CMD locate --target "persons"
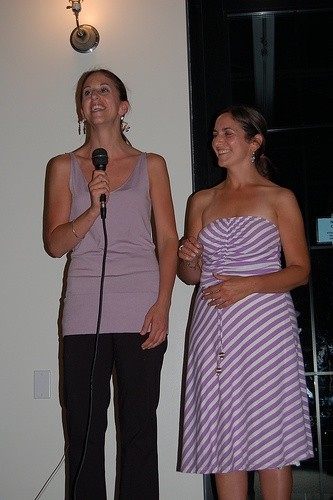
[43,69,181,500]
[176,107,314,500]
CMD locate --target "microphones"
[92,148,108,220]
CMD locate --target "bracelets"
[72,220,85,240]
[184,260,199,268]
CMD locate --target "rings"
[179,245,184,251]
